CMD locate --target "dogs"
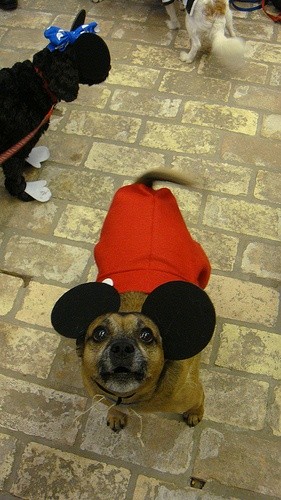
[0,32,111,202]
[51,170,216,432]
[162,0,244,64]
